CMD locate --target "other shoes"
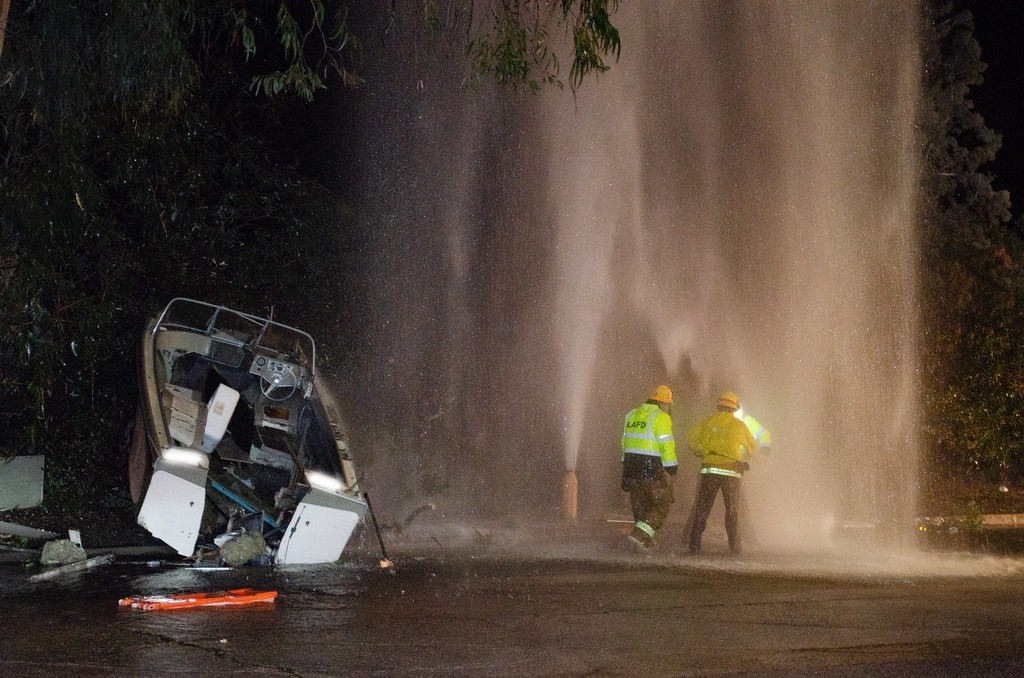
[626,535,650,554]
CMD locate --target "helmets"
[648,386,674,404]
[716,390,741,410]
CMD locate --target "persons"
[685,390,757,556]
[619,384,679,559]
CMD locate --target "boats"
[126,296,363,563]
